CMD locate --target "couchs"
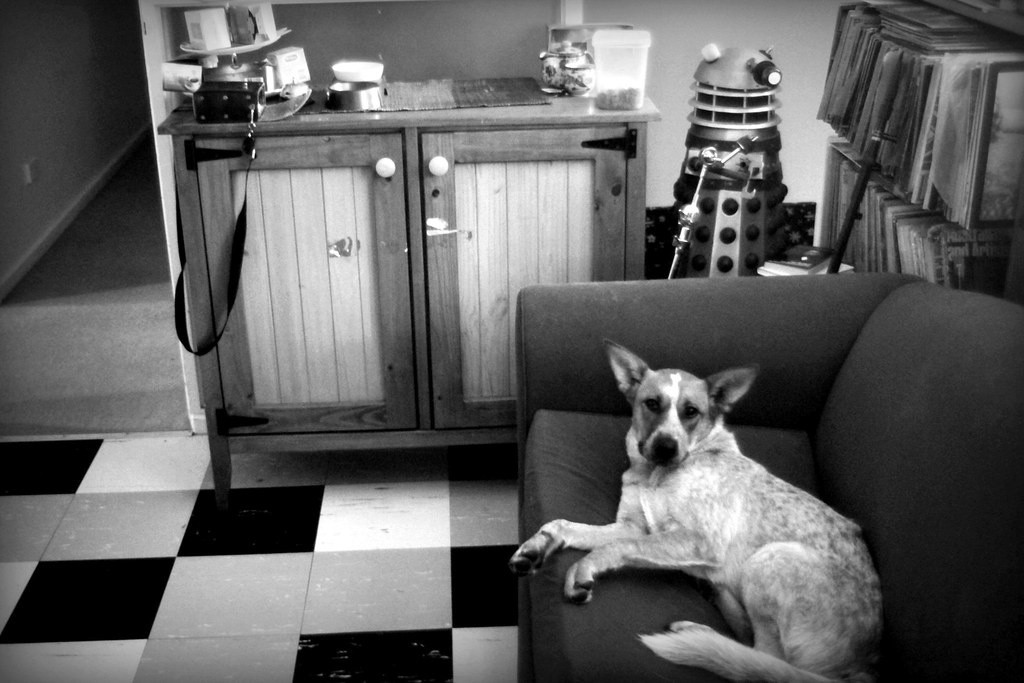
[511,278,1024,683]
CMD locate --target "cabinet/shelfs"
[158,76,660,486]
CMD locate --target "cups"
[564,62,596,97]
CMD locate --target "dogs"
[510,336,887,683]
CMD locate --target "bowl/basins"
[332,61,385,82]
[324,80,379,110]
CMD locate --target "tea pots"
[539,41,594,94]
